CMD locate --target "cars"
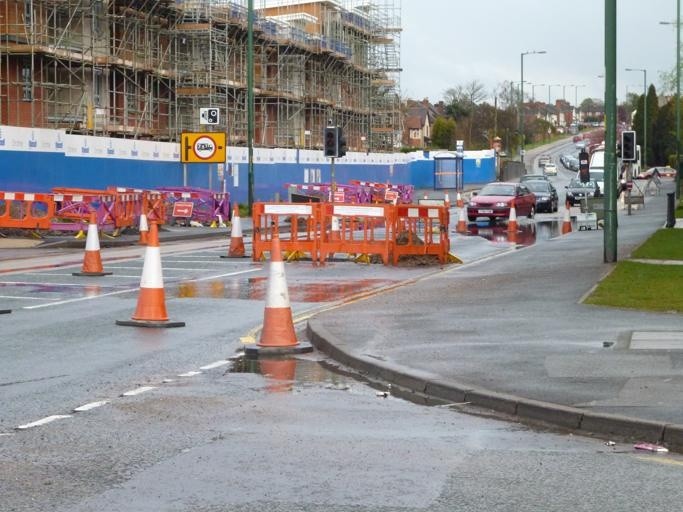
[465,179,537,223]
[589,120,605,128]
[465,219,538,250]
[518,131,676,216]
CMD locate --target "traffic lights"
[336,126,346,159]
[324,126,338,158]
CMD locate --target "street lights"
[656,20,680,163]
[624,68,649,171]
[507,79,589,144]
[596,74,605,79]
[518,49,551,161]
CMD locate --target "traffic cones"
[500,200,523,235]
[215,197,253,259]
[134,206,155,244]
[559,200,573,235]
[247,349,299,395]
[239,230,318,353]
[420,188,469,234]
[114,216,187,329]
[68,209,113,278]
